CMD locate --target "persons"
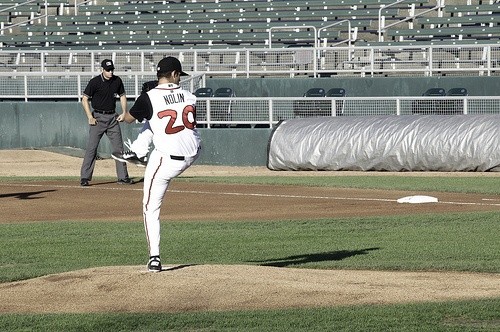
[112,57,201,273]
[81,58,134,186]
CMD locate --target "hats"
[102,59,114,72]
[156,56,189,78]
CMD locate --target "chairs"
[294,88,345,116]
[192,88,234,129]
[412,88,467,115]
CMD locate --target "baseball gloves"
[141,81,159,94]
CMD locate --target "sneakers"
[110,150,146,166]
[147,255,161,271]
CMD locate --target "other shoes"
[118,178,133,184]
[81,179,89,186]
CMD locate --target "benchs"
[0,0,500,78]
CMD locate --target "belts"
[96,110,113,114]
[170,156,185,160]
